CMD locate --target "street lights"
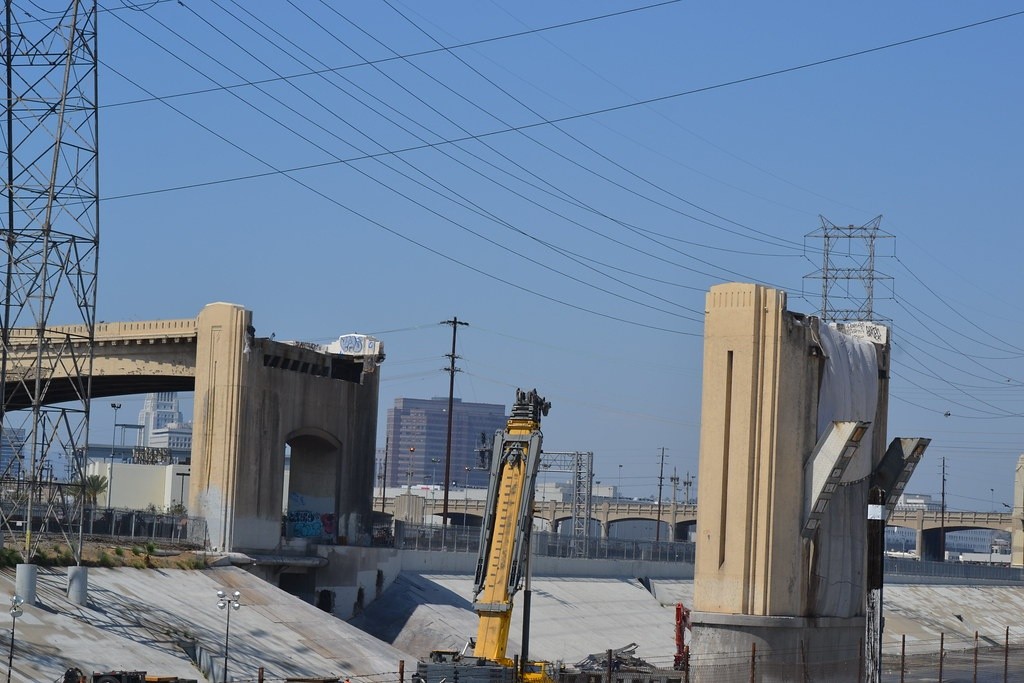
[464,466,471,553]
[990,489,994,512]
[617,465,623,503]
[428,458,441,551]
[594,479,599,558]
[407,447,415,522]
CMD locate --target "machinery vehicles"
[412,386,559,683]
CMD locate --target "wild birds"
[944,411,950,418]
[1002,503,1009,507]
